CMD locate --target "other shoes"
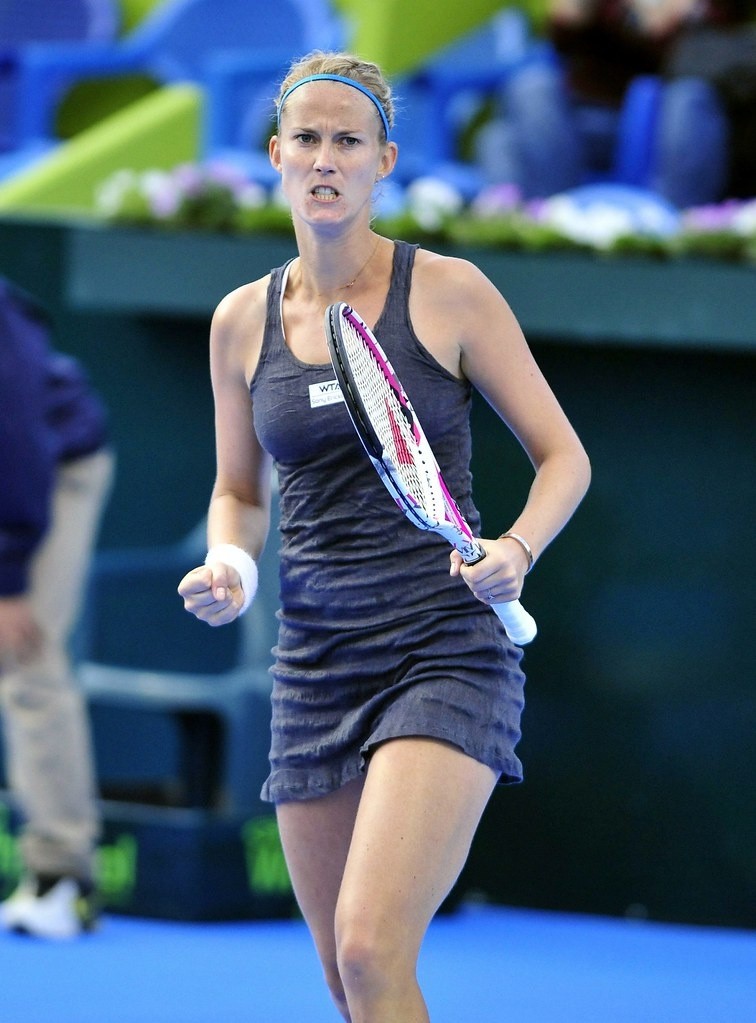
[0,870,85,938]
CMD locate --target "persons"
[0,284,121,940]
[177,55,593,1023]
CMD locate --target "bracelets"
[205,543,260,617]
[499,533,536,574]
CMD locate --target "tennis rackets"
[322,299,539,644]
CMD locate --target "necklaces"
[298,237,381,291]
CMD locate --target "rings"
[488,588,495,603]
